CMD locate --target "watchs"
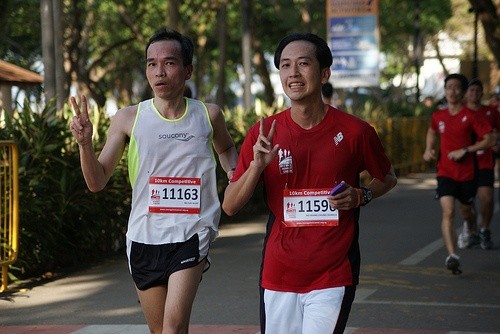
[360,186,373,207]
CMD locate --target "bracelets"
[354,188,360,208]
[463,147,469,153]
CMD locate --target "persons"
[322,82,340,110]
[463,78,500,251]
[222,34,397,334]
[423,73,493,275]
[69,26,239,334]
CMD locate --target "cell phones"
[330,183,346,197]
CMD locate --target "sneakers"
[446,254,464,276]
[480,227,492,249]
[457,220,474,250]
